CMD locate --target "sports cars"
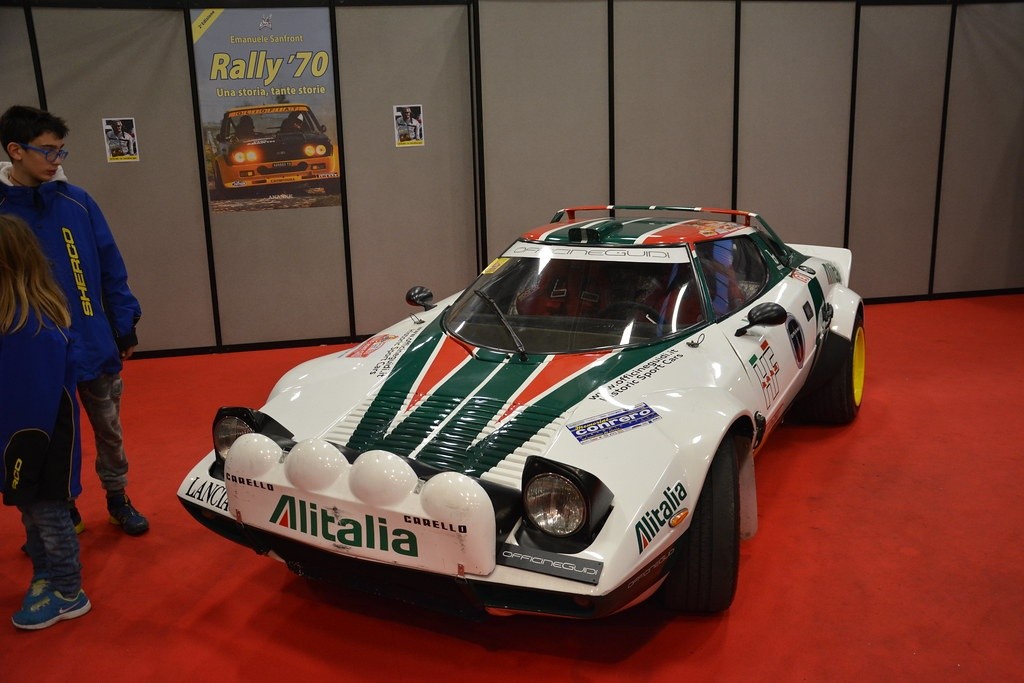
[179,205,865,629]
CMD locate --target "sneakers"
[20,573,49,609]
[106,493,148,533]
[21,508,85,553]
[11,590,91,631]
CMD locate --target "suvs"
[206,102,342,197]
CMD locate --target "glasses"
[20,145,68,162]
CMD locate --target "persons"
[0,214,92,629]
[396,107,420,141]
[280,111,305,134]
[106,120,134,157]
[0,105,150,558]
[234,115,256,141]
